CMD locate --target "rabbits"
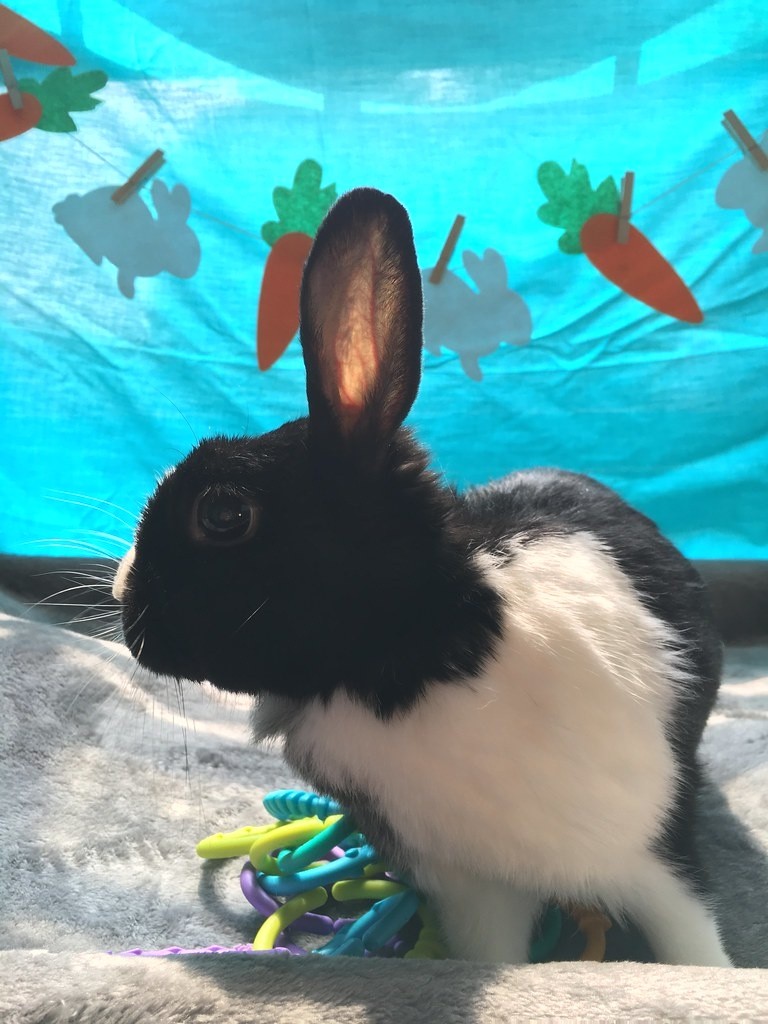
[101,188,738,968]
